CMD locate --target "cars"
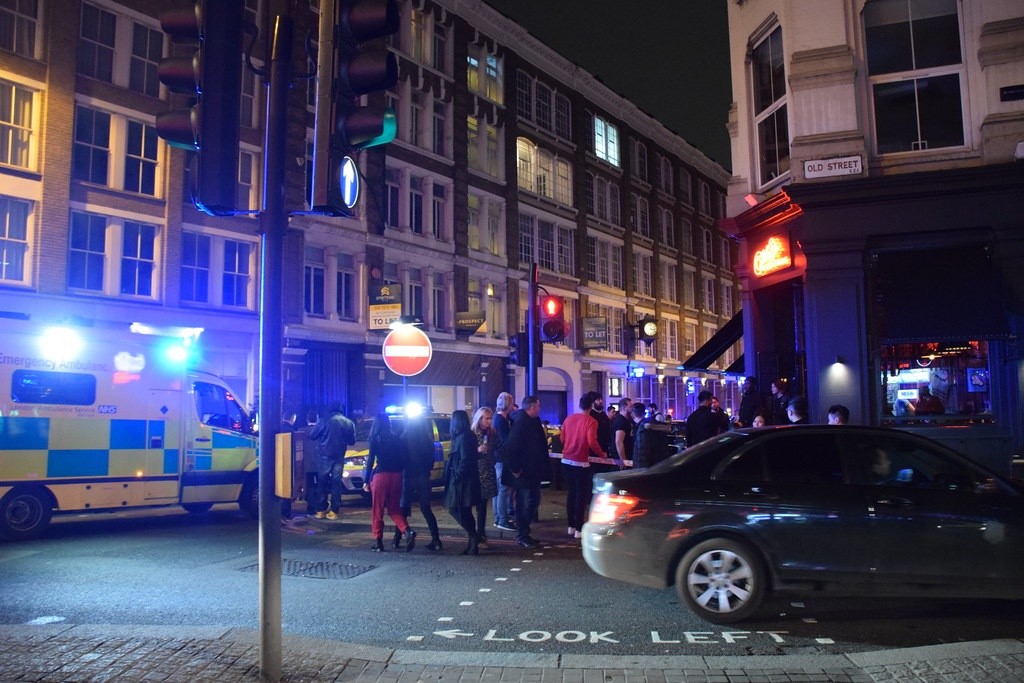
[340,413,452,505]
[581,423,1024,626]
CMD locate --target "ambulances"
[0,332,260,541]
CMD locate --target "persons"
[895,392,915,416]
[767,379,794,425]
[738,376,764,428]
[786,399,808,424]
[441,391,551,557]
[560,396,607,539]
[687,391,729,448]
[750,408,773,428]
[826,405,850,425]
[362,409,443,552]
[278,400,356,519]
[915,386,944,415]
[584,391,671,509]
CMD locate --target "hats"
[588,391,602,403]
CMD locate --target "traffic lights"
[541,294,566,343]
[157,0,244,217]
[326,0,399,220]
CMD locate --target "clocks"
[644,322,657,336]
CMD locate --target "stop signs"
[382,326,432,377]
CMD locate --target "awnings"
[676,308,745,377]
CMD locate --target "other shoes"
[406,530,416,551]
[371,543,383,551]
[519,540,539,548]
[567,527,576,535]
[425,540,442,550]
[326,512,336,519]
[574,530,581,539]
[494,522,519,531]
[391,541,398,549]
[285,517,295,522]
[315,511,323,519]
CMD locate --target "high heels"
[459,544,479,554]
[469,531,485,552]
